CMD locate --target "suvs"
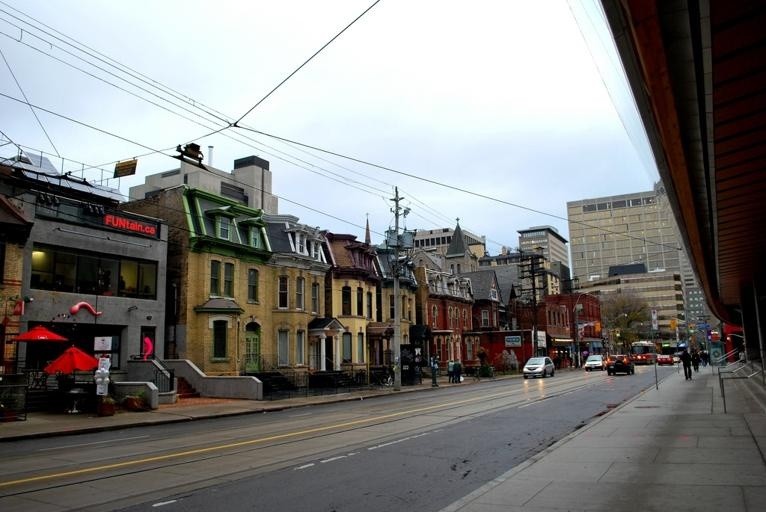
[607,355,634,376]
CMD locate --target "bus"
[699,343,708,354]
[629,338,689,366]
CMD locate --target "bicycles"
[379,370,394,387]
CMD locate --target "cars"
[584,355,606,371]
[523,357,555,378]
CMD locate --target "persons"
[553,354,587,371]
[448,355,481,383]
[680,348,708,380]
[739,350,745,366]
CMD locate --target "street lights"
[614,313,627,322]
[570,290,591,367]
[392,247,437,390]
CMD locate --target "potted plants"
[98,397,116,415]
[2,390,25,421]
[126,388,146,408]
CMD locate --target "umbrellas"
[44,347,99,389]
[12,324,69,370]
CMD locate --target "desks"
[20,367,88,413]
